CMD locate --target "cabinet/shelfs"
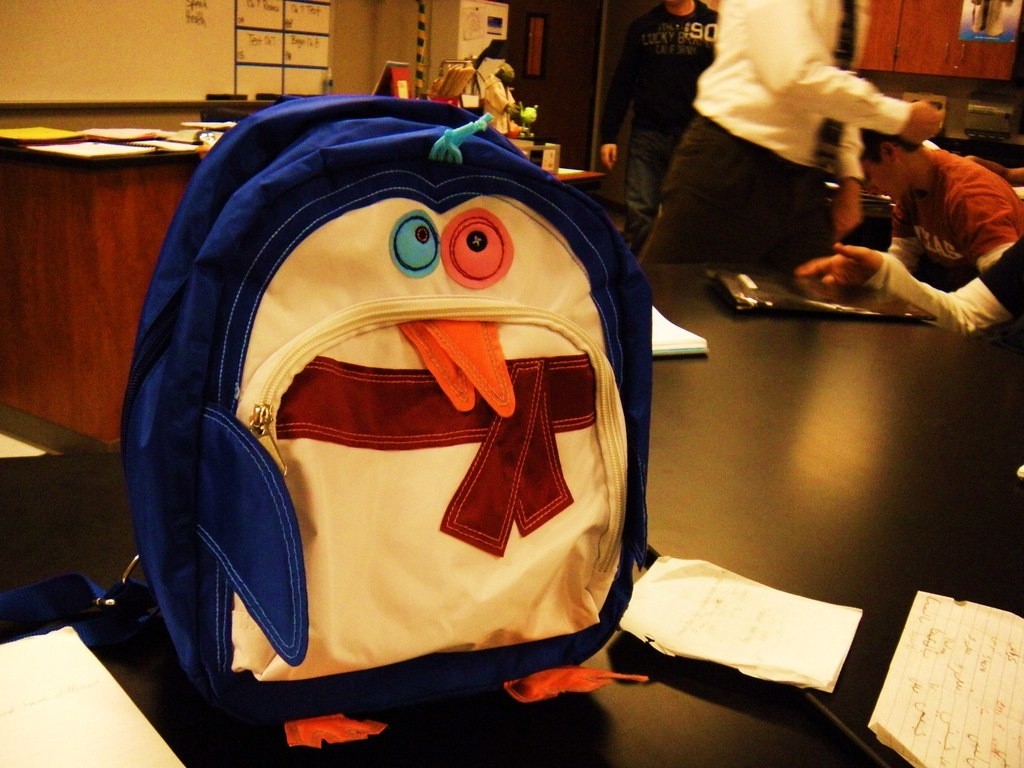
[858,0,1023,81]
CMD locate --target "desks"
[0,140,208,456]
[0,264,1024,768]
[534,166,604,183]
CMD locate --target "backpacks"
[0,97,651,746]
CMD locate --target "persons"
[600,0,719,252]
[484,63,521,134]
[795,128,1024,341]
[639,1,944,266]
